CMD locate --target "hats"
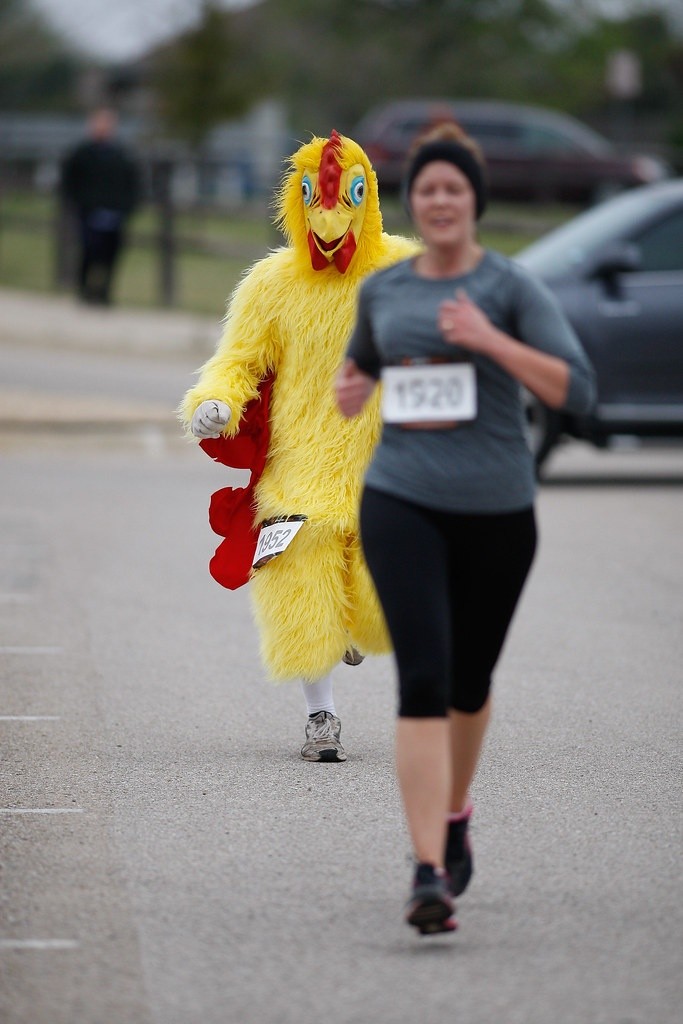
[401,120,490,220]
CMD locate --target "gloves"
[189,398,231,439]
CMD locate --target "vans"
[511,180,683,470]
[355,100,665,211]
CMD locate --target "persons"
[333,134,592,935]
[409,112,489,220]
[57,109,145,305]
[181,130,423,760]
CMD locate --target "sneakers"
[301,711,346,762]
[445,797,472,897]
[342,641,365,666]
[401,863,457,934]
